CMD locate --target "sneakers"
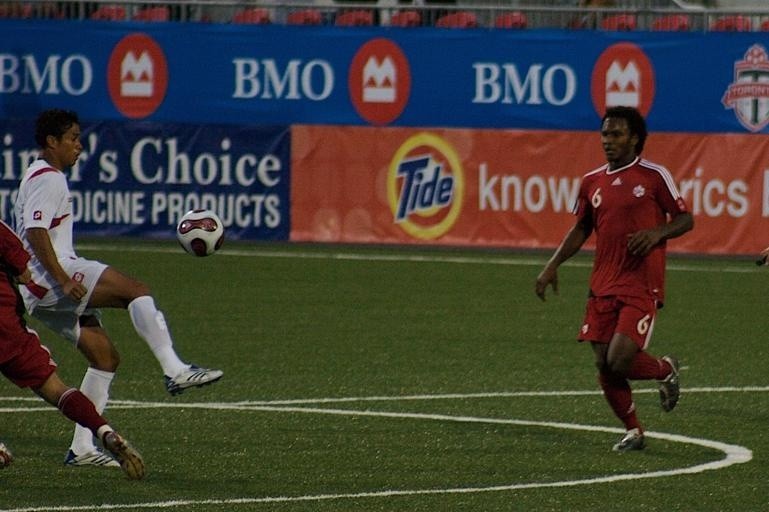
[102,430,145,479]
[613,431,646,451]
[658,354,680,412]
[63,449,122,468]
[164,365,224,395]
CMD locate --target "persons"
[536,104,694,453]
[0,217,146,479]
[17,108,224,469]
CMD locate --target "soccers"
[176,208,225,257]
